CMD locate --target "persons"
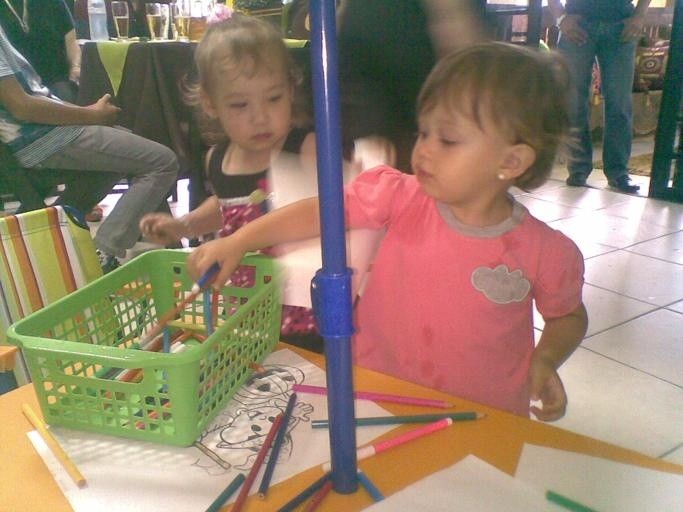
[546,1,655,194]
[336,0,493,175]
[171,36,592,429]
[0,26,180,272]
[0,0,85,91]
[133,13,325,352]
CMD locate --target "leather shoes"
[607,176,640,192]
[565,171,588,187]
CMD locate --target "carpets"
[592,147,682,183]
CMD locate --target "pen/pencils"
[87,262,265,432]
[203,385,488,512]
[21,402,86,488]
[546,490,595,512]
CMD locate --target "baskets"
[4,247,282,449]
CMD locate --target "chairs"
[1,142,124,391]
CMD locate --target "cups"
[174,0,215,43]
[110,2,130,40]
[145,3,170,41]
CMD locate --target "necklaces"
[4,1,29,32]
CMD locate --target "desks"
[77,39,311,165]
[0,312,683,511]
